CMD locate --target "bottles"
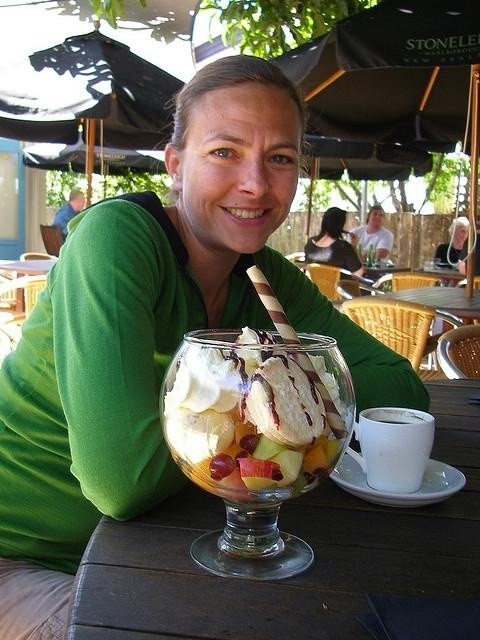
[357,240,376,268]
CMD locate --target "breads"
[234,327,326,447]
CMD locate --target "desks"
[297,261,374,307]
[380,283,477,319]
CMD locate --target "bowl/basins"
[419,257,442,267]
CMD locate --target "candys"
[246,264,348,439]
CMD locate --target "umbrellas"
[266,0,480,324]
[1,20,186,207]
[21,141,167,199]
[299,138,434,233]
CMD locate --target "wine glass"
[157,327,360,584]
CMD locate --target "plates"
[329,449,466,509]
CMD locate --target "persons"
[448,233,480,289]
[52,191,85,240]
[304,207,364,289]
[346,206,394,295]
[0,54,430,640]
[434,217,469,286]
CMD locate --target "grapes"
[209,454,235,478]
[240,435,259,452]
[235,450,251,471]
[313,467,328,480]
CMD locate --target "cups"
[346,406,435,495]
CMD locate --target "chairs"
[329,276,386,301]
[436,322,480,377]
[338,292,462,378]
[0,224,65,337]
[375,270,444,298]
